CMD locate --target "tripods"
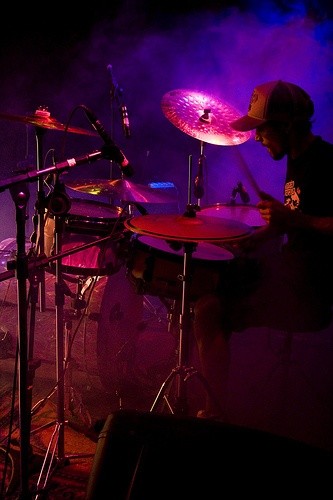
[149,244,227,419]
[30,212,100,500]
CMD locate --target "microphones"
[133,203,181,252]
[51,152,60,188]
[81,105,136,177]
[194,141,206,198]
[120,105,134,136]
[236,181,250,202]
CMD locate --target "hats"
[230,80,314,132]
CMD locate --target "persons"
[195,81,332,419]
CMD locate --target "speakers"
[87,411,333,500]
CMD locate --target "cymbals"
[160,89,250,146]
[123,213,255,243]
[65,176,179,205]
[0,108,98,138]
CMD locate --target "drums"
[126,235,234,297]
[0,236,146,395]
[34,205,124,275]
[198,202,274,235]
